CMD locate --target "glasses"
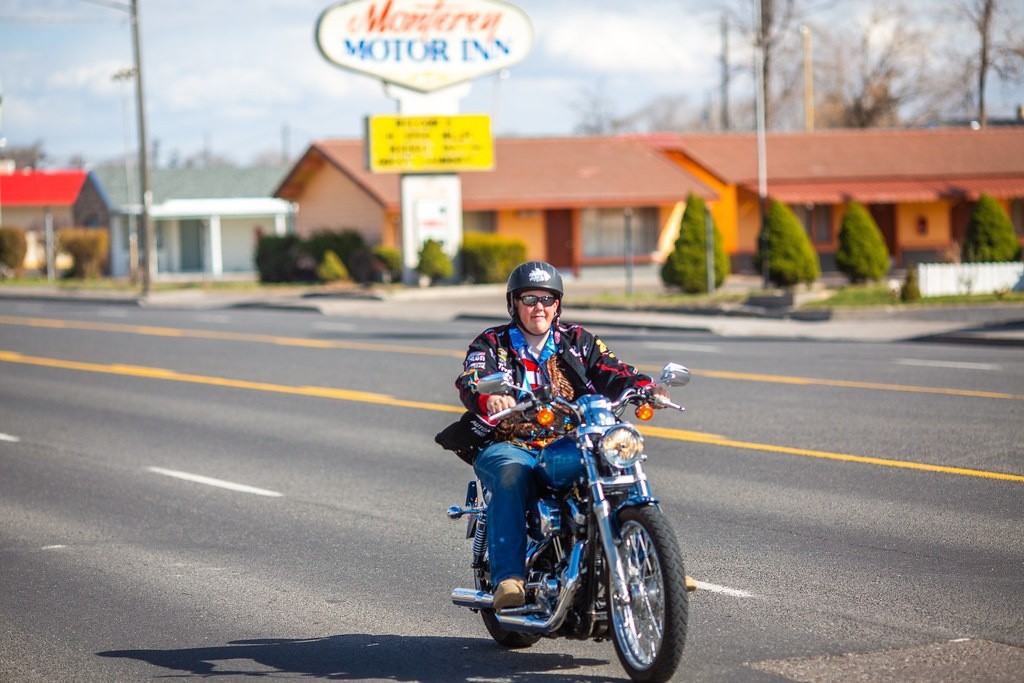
[514,294,556,307]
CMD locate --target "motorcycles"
[445,361,696,683]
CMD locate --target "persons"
[454,261,670,610]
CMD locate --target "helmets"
[506,261,563,303]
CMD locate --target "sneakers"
[494,577,527,611]
[685,575,697,592]
